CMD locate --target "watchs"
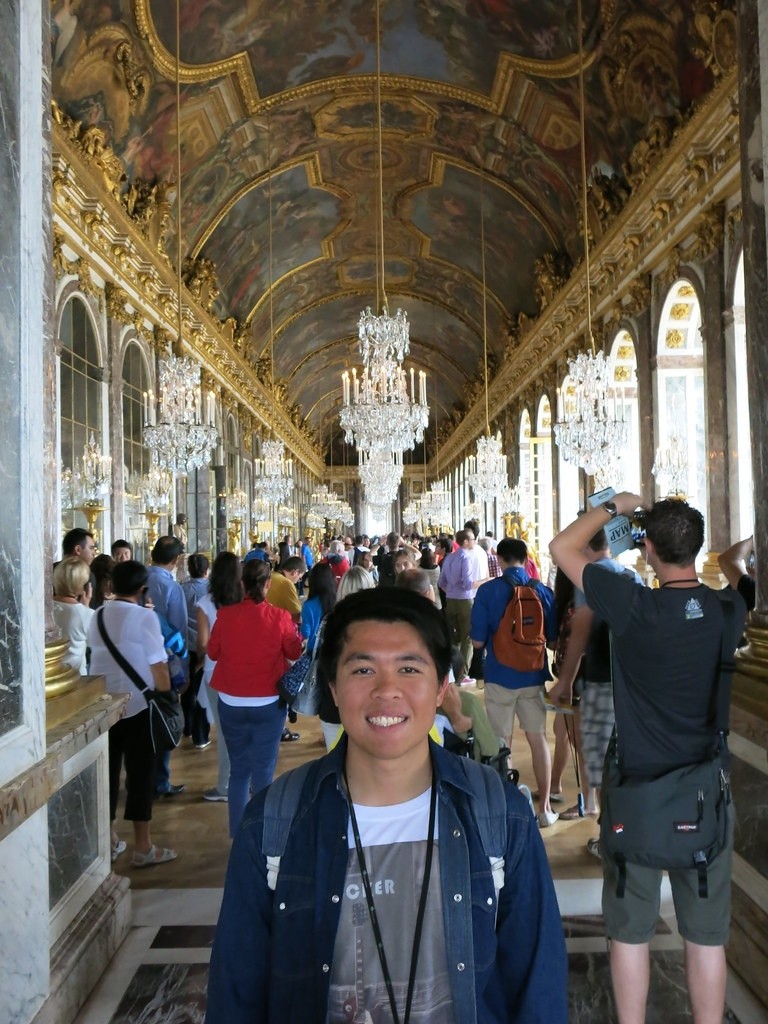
[603,500,617,520]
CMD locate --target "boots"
[453,665,476,686]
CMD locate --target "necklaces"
[114,597,134,603]
[661,578,698,586]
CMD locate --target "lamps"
[550,1,632,479]
[139,465,170,561]
[648,436,694,502]
[224,0,533,554]
[73,437,112,550]
[136,0,219,476]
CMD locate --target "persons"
[207,584,574,1024]
[54,492,756,860]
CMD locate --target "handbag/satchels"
[596,760,734,872]
[279,656,320,718]
[146,693,186,752]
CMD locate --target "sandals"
[111,840,127,864]
[132,845,178,867]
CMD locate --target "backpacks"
[495,576,548,672]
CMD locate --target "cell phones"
[630,511,647,550]
[142,586,149,608]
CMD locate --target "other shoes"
[560,804,599,820]
[156,784,184,799]
[281,730,301,742]
[203,788,228,802]
[588,837,602,858]
[196,740,213,749]
[540,808,560,827]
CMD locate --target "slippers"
[530,790,564,802]
